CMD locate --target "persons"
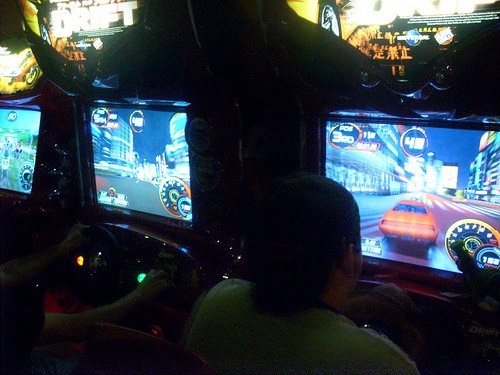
[184,174,500,375]
[0,223,167,375]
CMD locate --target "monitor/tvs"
[0,105,46,200]
[80,99,199,232]
[319,115,500,287]
